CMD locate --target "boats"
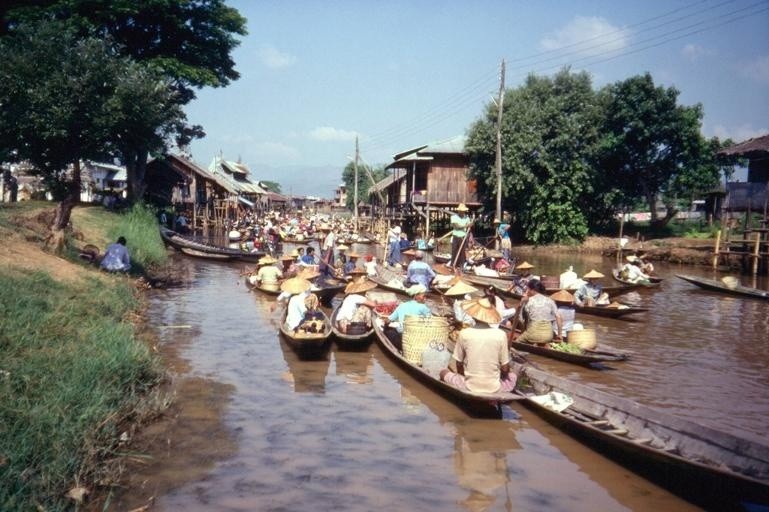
[246,213,650,319]
[279,305,333,350]
[158,222,267,261]
[672,273,769,301]
[499,322,632,366]
[510,363,769,495]
[612,268,663,289]
[329,301,378,344]
[369,313,529,409]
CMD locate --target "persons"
[403,251,436,291]
[440,298,517,392]
[551,290,576,337]
[229,204,356,252]
[494,218,511,260]
[445,276,506,329]
[384,284,431,350]
[99,236,131,273]
[623,250,654,282]
[517,278,562,348]
[573,269,605,307]
[510,261,534,295]
[428,235,434,247]
[451,205,472,272]
[256,223,379,332]
[160,210,167,222]
[386,222,410,266]
[176,215,188,225]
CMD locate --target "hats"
[455,203,470,211]
[583,269,606,279]
[493,218,502,224]
[515,261,535,269]
[487,249,505,258]
[460,298,501,323]
[401,248,479,296]
[258,223,378,294]
[626,253,651,263]
[549,289,578,302]
